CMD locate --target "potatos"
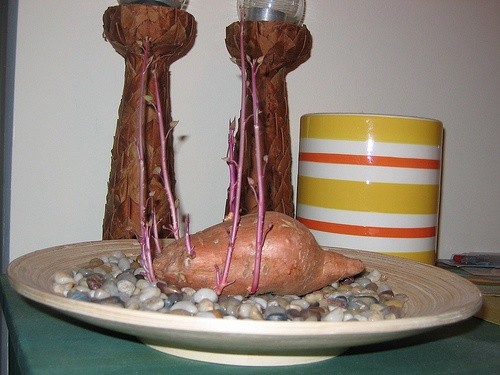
[153,211,366,296]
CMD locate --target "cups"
[295,114,443,267]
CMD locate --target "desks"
[1,274,499,375]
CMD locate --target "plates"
[8,239,483,368]
